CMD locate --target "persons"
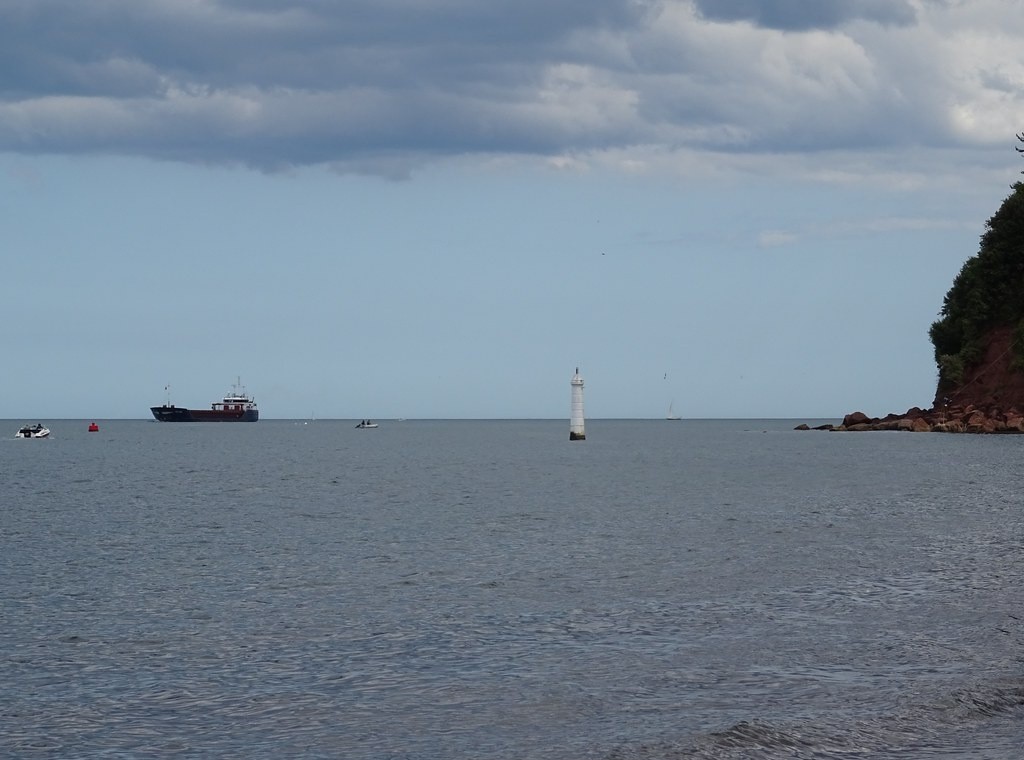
[24,423,44,430]
[361,418,371,425]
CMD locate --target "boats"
[357,424,378,429]
[14,423,50,438]
[150,376,259,422]
